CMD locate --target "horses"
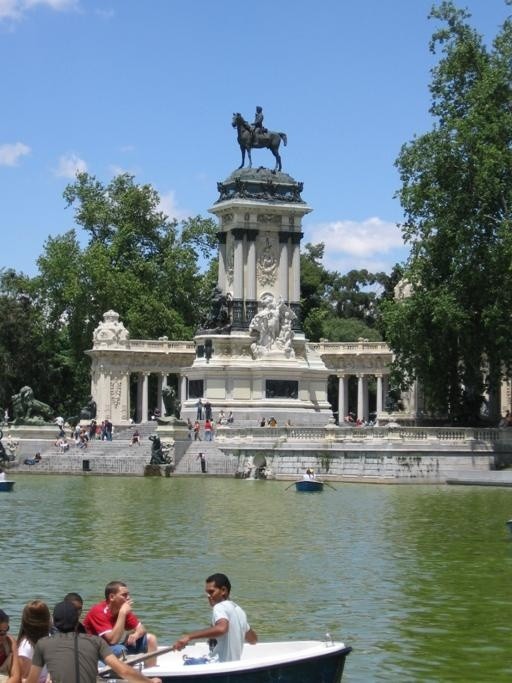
[231,112,288,172]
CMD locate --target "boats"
[97,632,352,682]
[295,479,324,490]
[0,479,15,490]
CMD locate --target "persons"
[331,409,386,429]
[18,600,51,682]
[267,416,277,426]
[63,591,85,632]
[304,465,316,482]
[22,599,163,682]
[194,452,208,473]
[248,105,267,146]
[24,450,43,464]
[154,409,161,419]
[83,579,159,678]
[186,397,235,443]
[258,415,265,428]
[284,418,296,431]
[248,294,298,352]
[48,415,115,451]
[1,607,31,682]
[171,572,258,668]
[148,433,172,464]
[129,429,142,448]
